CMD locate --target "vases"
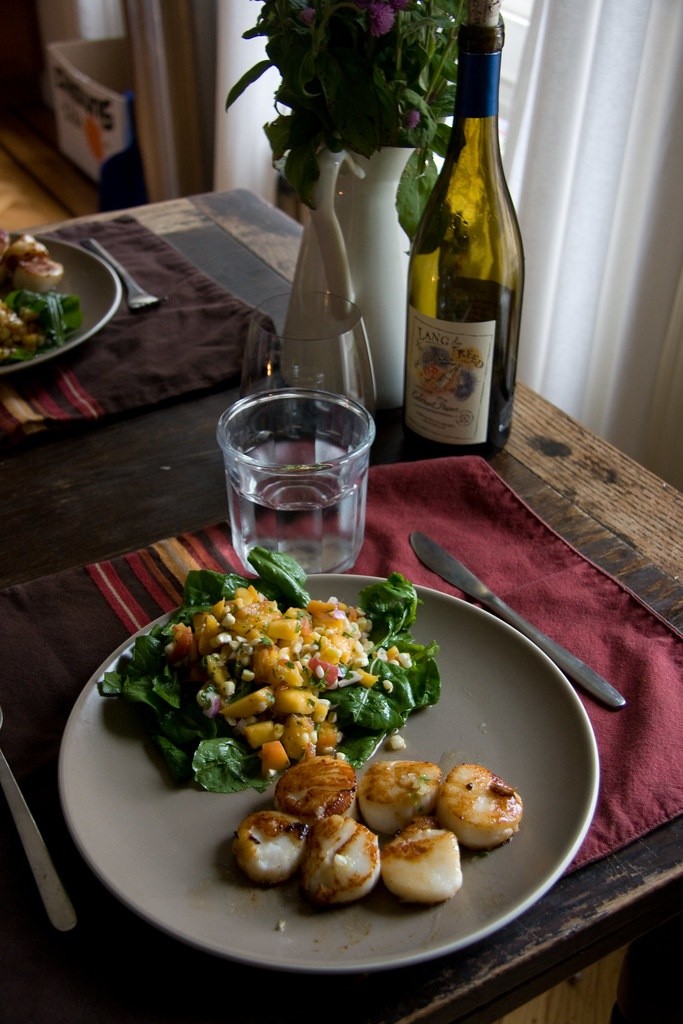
[282,144,413,410]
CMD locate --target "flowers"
[225,1,476,260]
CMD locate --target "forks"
[79,238,169,311]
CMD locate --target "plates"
[0,231,124,373]
[58,570,602,974]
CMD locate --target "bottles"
[399,0,525,456]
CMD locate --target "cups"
[216,385,376,577]
[243,291,379,422]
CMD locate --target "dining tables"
[1,188,683,1023]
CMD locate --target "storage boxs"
[48,34,135,183]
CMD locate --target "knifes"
[398,529,627,702]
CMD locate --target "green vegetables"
[0,287,84,362]
[97,546,441,791]
